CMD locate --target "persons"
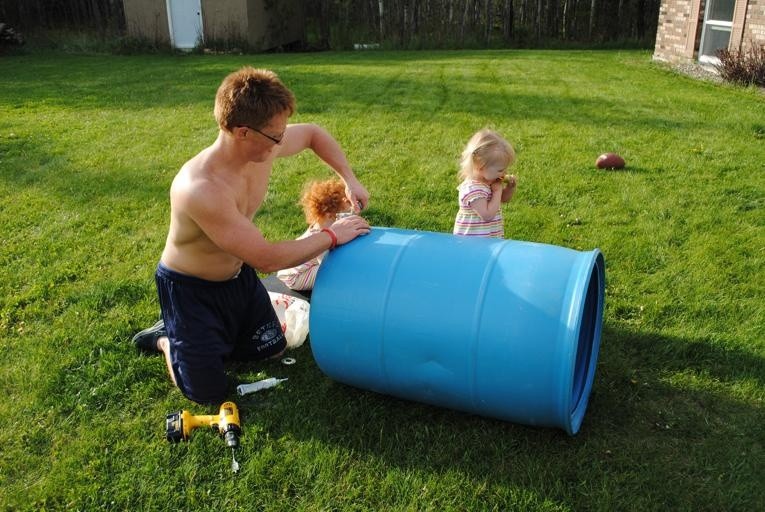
[277,178,350,291]
[451,128,518,238]
[130,67,371,403]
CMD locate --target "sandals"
[131,319,168,356]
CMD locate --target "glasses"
[237,124,286,145]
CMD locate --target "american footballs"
[596,153,625,168]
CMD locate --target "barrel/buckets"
[308,226,605,437]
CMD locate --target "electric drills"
[166,402,242,475]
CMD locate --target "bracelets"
[321,227,337,250]
